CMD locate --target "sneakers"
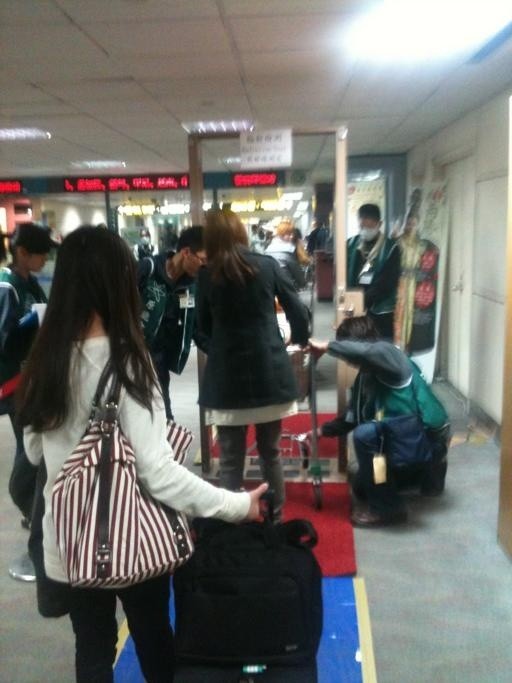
[8,551,36,584]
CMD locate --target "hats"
[13,221,61,255]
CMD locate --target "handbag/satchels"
[27,457,73,619]
[372,410,433,474]
[47,344,195,589]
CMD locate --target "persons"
[0,222,58,530]
[391,188,439,358]
[190,208,314,526]
[10,224,270,683]
[248,214,328,296]
[132,228,155,259]
[127,225,209,422]
[302,316,453,526]
[346,202,400,332]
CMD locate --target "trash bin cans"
[313,250,334,303]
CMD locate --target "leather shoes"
[350,501,409,529]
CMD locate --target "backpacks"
[177,514,324,663]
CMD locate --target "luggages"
[169,631,320,683]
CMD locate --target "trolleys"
[195,339,323,510]
